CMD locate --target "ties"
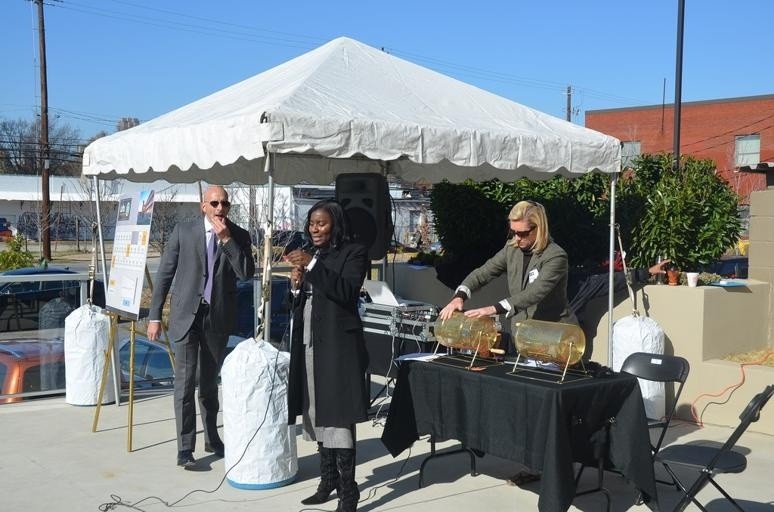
[203,229,216,305]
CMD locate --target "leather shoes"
[178,450,196,466]
[205,444,224,457]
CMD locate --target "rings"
[295,272,296,273]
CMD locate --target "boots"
[334,448,360,512]
[301,446,340,504]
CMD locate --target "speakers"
[336,173,394,260]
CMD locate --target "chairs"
[575,352,690,505]
[654,385,773,511]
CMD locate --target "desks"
[381,353,657,511]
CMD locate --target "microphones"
[295,264,304,289]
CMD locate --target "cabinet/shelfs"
[92,190,176,452]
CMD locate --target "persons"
[147,186,255,467]
[287,199,369,512]
[552,225,670,314]
[439,200,580,486]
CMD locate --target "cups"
[686,266,700,287]
[655,255,664,285]
[666,264,680,286]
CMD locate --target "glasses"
[210,201,230,207]
[509,224,535,237]
